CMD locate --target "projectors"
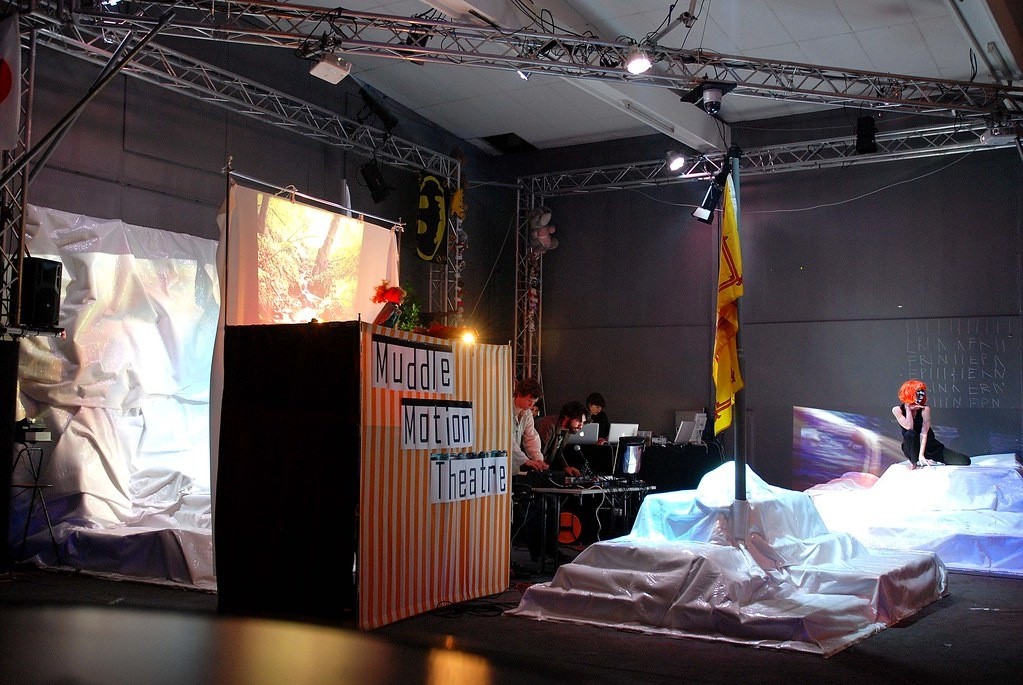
[309,52,352,85]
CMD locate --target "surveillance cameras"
[703,88,722,115]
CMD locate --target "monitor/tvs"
[612,436,648,486]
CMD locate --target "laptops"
[673,421,696,443]
[568,423,600,444]
[608,423,639,445]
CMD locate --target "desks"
[530,483,660,577]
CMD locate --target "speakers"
[9,256,61,327]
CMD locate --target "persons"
[511,378,550,475]
[891,379,971,470]
[535,400,590,477]
[373,281,407,327]
[584,392,610,445]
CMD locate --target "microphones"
[574,444,589,463]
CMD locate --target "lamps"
[667,150,686,171]
[628,43,652,74]
[517,69,531,81]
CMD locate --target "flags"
[711,175,744,435]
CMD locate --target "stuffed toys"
[528,255,539,317]
[451,188,469,323]
[530,205,560,254]
